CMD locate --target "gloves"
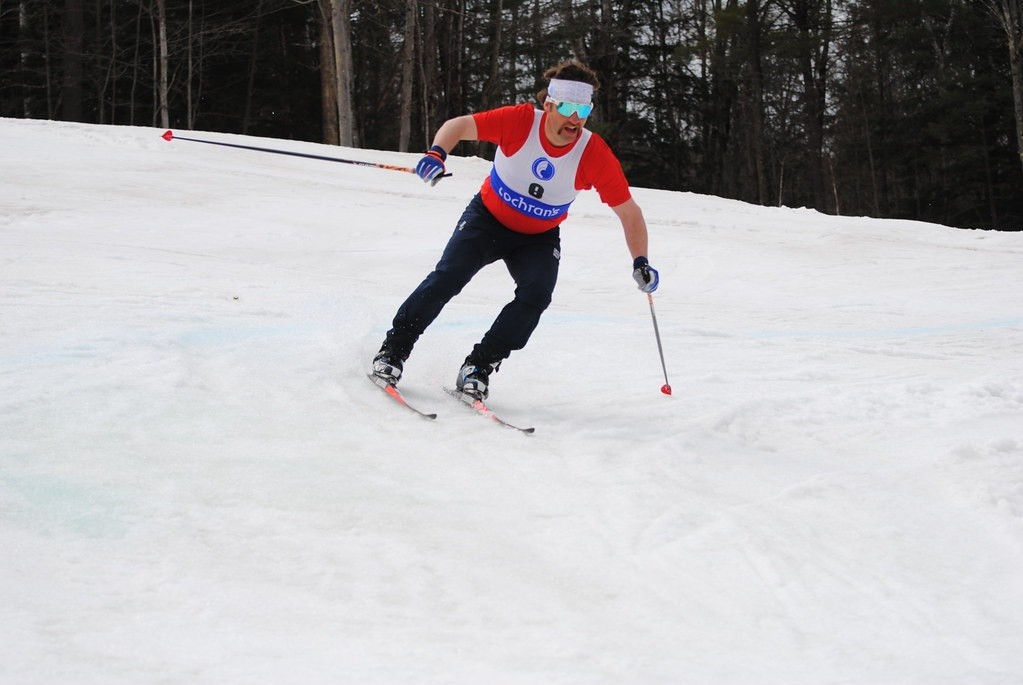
[415,144,447,187]
[632,260,660,292]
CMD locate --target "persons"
[370,59,659,400]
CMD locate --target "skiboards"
[366,373,536,434]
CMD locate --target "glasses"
[556,101,592,119]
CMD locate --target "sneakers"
[372,337,412,386]
[455,343,503,402]
